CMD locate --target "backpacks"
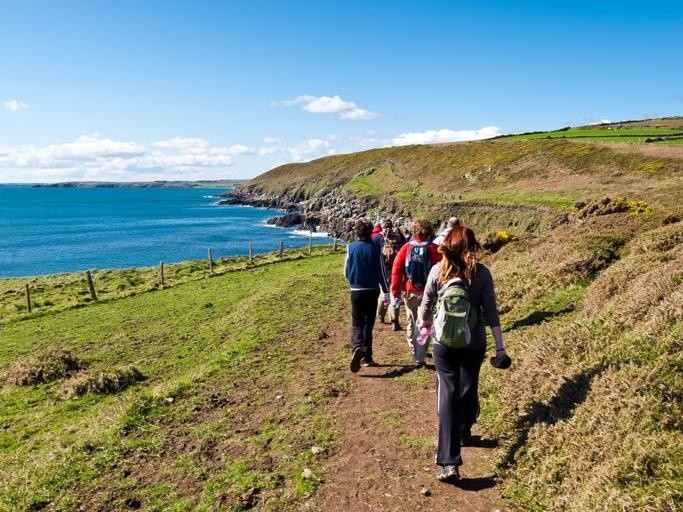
[430,277,477,349]
[404,240,434,289]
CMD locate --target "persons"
[343,219,390,372]
[417,225,507,480]
[371,216,460,364]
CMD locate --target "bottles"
[416,325,430,346]
[393,297,401,309]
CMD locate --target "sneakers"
[391,322,402,331]
[435,464,460,480]
[349,346,426,372]
[460,431,472,446]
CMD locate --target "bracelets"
[496,348,504,351]
